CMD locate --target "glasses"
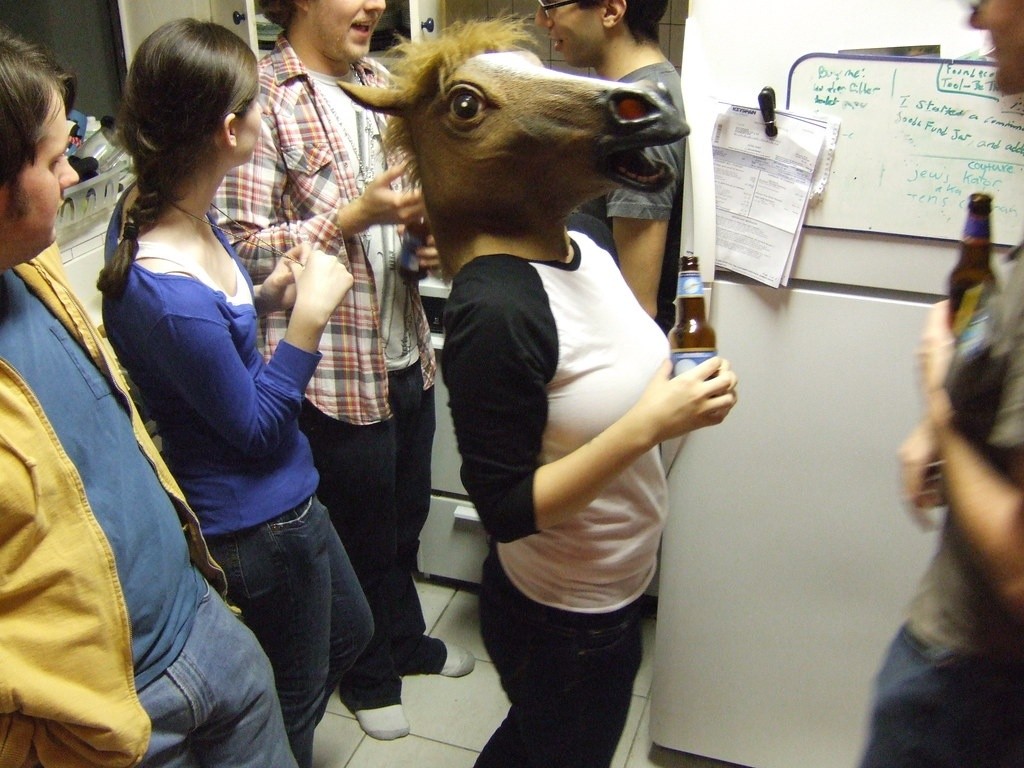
[539,0,584,18]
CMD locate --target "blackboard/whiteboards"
[787,53,1024,248]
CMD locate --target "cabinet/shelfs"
[208,0,446,66]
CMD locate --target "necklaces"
[173,203,306,271]
[311,63,376,193]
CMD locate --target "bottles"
[669,254,721,382]
[398,210,432,280]
[949,194,998,346]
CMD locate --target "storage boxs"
[54,158,127,247]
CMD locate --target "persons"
[853,3,1023,768]
[336,7,737,768]
[0,29,300,768]
[208,0,475,743]
[95,18,373,768]
[533,0,688,336]
